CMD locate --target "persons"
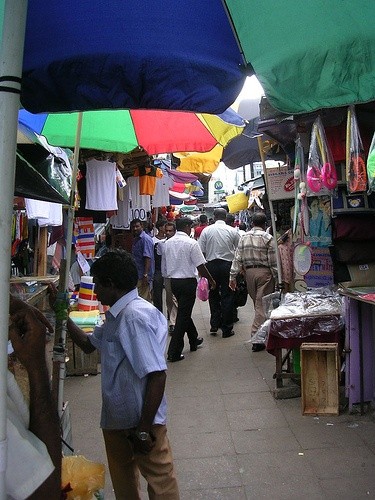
[308,196,332,248]
[194,215,209,239]
[197,208,241,338]
[165,217,217,362]
[0,292,62,500]
[130,218,154,303]
[238,222,246,236]
[156,222,179,336]
[49,249,179,499]
[152,220,170,320]
[225,214,235,227]
[229,212,277,352]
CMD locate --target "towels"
[25,198,48,219]
[38,203,62,227]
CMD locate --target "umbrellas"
[171,180,204,193]
[155,159,210,183]
[168,190,196,206]
[222,98,295,170]
[14,108,247,418]
[0,0,375,500]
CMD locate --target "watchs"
[135,430,150,441]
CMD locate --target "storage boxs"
[300,342,341,418]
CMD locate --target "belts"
[245,264,270,270]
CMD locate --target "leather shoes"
[252,344,266,352]
[191,337,203,351]
[168,355,184,362]
[169,325,175,334]
[210,328,216,332]
[223,331,234,337]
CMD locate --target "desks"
[267,315,342,387]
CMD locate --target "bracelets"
[209,278,214,283]
[143,274,147,277]
